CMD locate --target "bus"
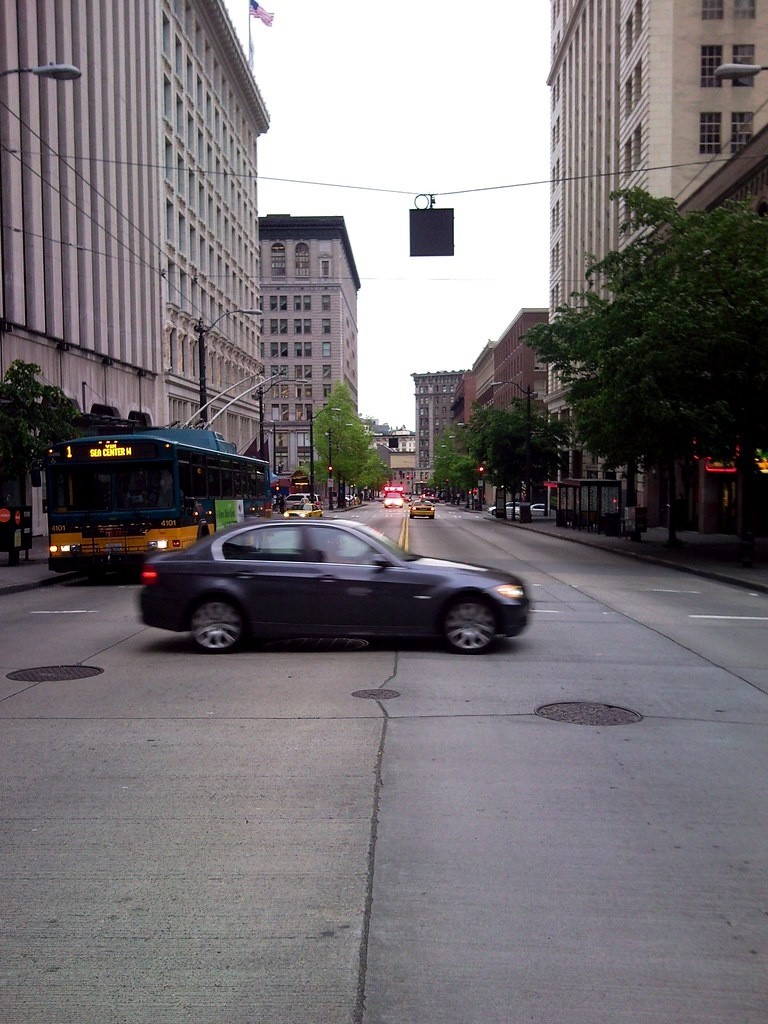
[29,427,272,574]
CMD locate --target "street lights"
[325,423,353,511]
[193,309,264,429]
[490,381,538,503]
[255,378,309,461]
[456,422,484,511]
[309,408,341,503]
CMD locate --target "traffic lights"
[328,465,333,474]
[478,465,484,473]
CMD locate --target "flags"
[249,0,274,27]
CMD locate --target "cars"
[282,497,323,518]
[486,501,556,520]
[382,485,437,508]
[137,515,531,655]
[408,499,436,519]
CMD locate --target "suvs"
[284,493,318,510]
